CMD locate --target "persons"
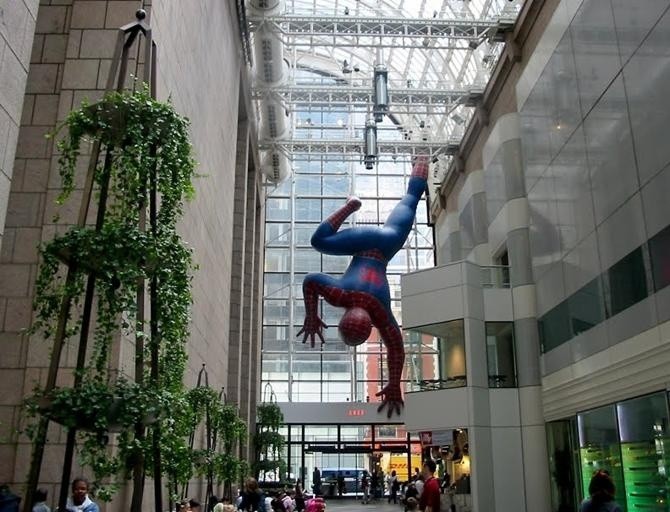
[176,456,470,512]
[578,471,622,511]
[67,475,100,512]
[30,487,52,511]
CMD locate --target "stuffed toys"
[296,151,433,418]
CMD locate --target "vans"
[318,468,372,495]
